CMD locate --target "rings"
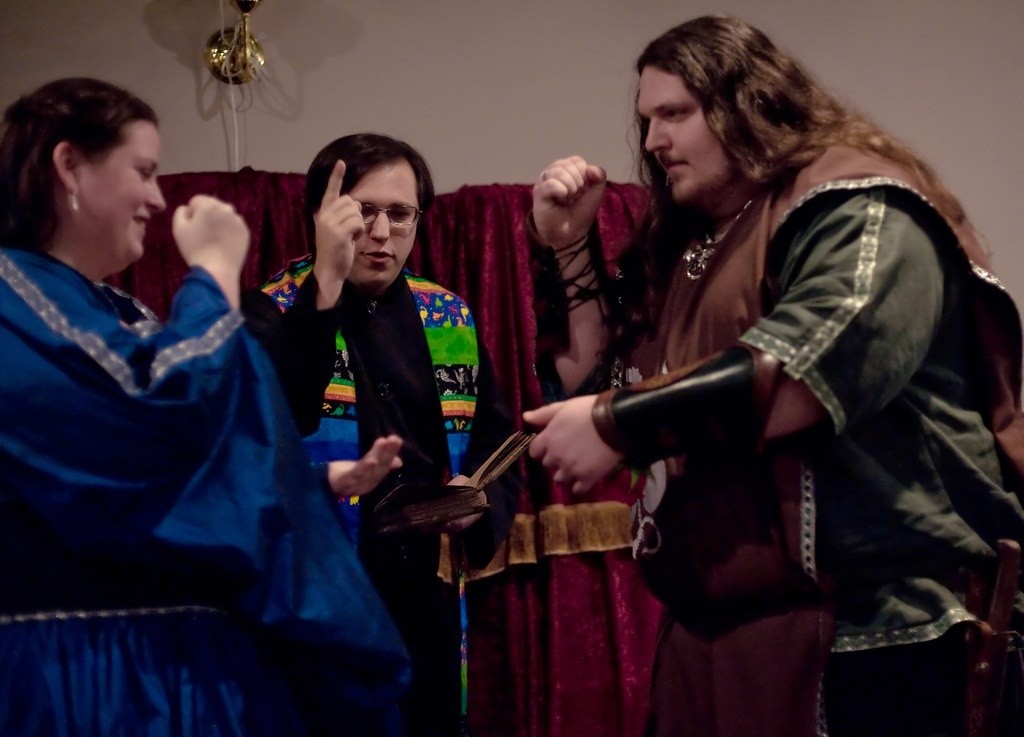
[542,169,548,181]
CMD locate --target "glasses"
[360,205,424,224]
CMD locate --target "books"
[374,431,536,533]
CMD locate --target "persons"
[0,77,402,737]
[243,134,515,737]
[522,15,1024,737]
